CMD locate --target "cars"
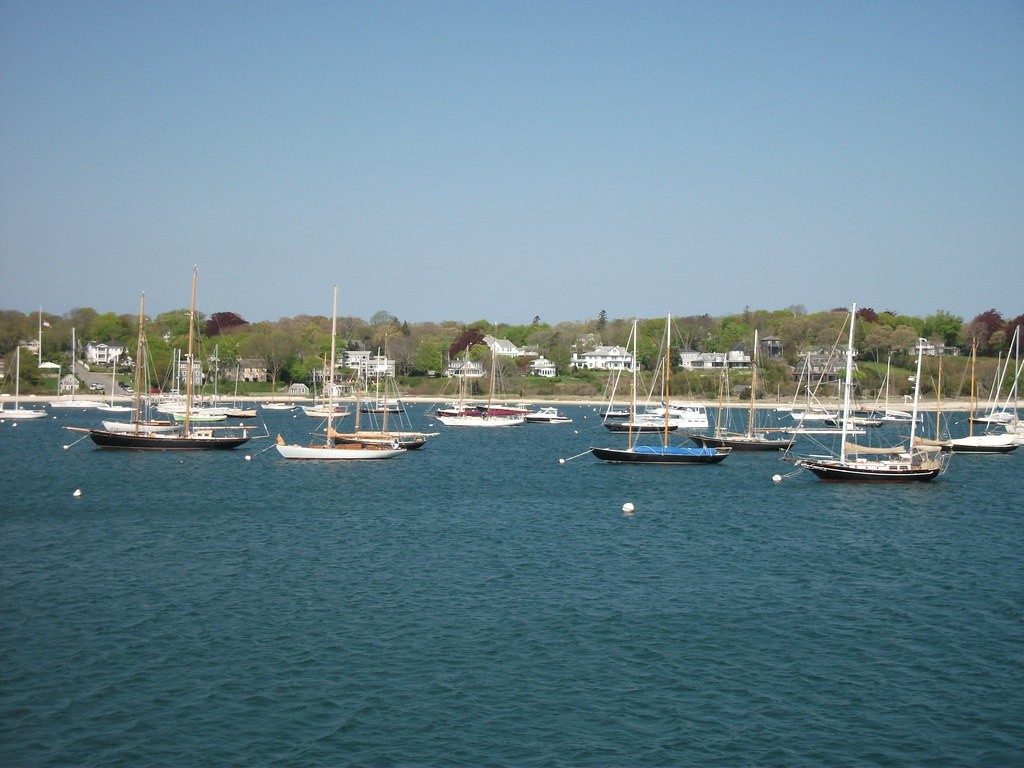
[123,385,129,390]
[126,387,134,393]
[147,388,161,393]
[118,382,125,388]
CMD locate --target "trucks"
[90,384,106,391]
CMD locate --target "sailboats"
[557,305,734,466]
[0,345,48,419]
[423,319,574,429]
[60,262,353,452]
[329,332,441,452]
[275,284,408,461]
[595,302,1024,484]
